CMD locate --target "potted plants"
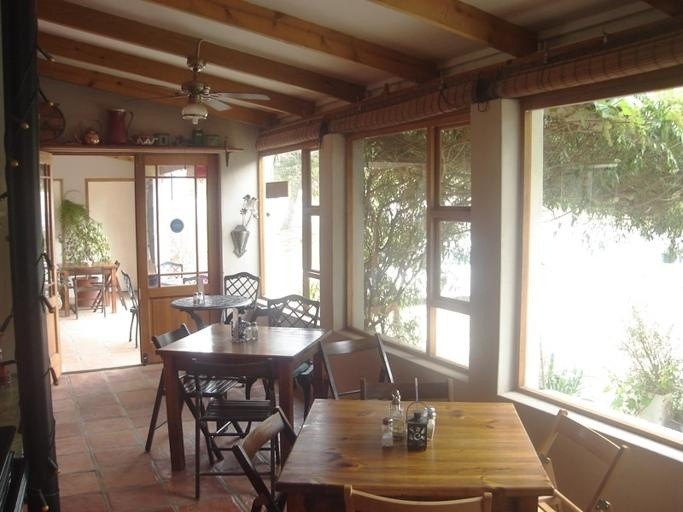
[58,199,111,307]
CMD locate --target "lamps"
[182,94,207,124]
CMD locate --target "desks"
[55,262,118,312]
[169,292,253,330]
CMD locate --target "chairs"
[233,404,300,511]
[190,357,280,501]
[358,375,460,405]
[244,294,322,421]
[537,404,628,511]
[156,263,209,285]
[316,329,395,400]
[222,269,263,325]
[55,264,79,318]
[146,321,240,466]
[343,484,496,510]
[89,260,127,312]
[121,270,139,348]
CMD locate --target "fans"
[135,40,269,112]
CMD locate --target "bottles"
[230,307,258,342]
[383,389,436,450]
[193,292,203,305]
